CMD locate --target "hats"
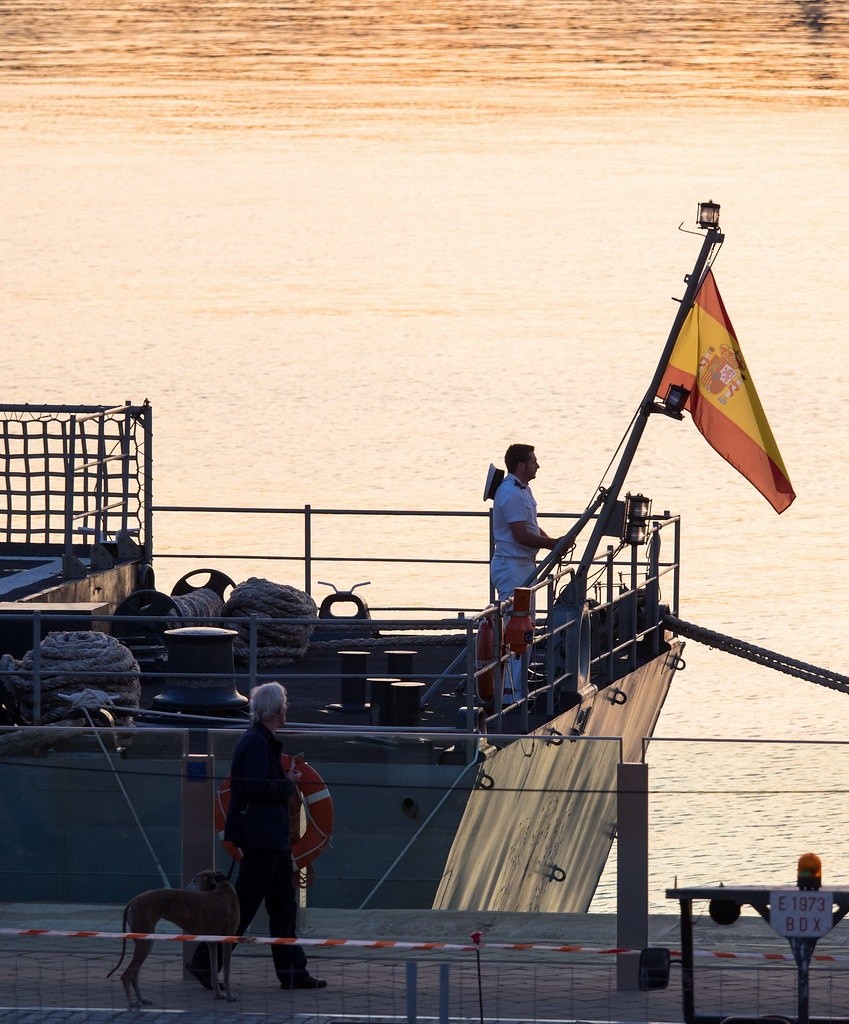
[482,463,504,502]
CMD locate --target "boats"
[1,198,732,912]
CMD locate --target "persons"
[180,681,328,992]
[489,444,566,712]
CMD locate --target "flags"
[656,265,797,516]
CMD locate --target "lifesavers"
[476,617,507,703]
[210,753,333,871]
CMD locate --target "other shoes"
[185,960,225,992]
[280,968,328,990]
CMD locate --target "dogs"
[105,871,240,1006]
